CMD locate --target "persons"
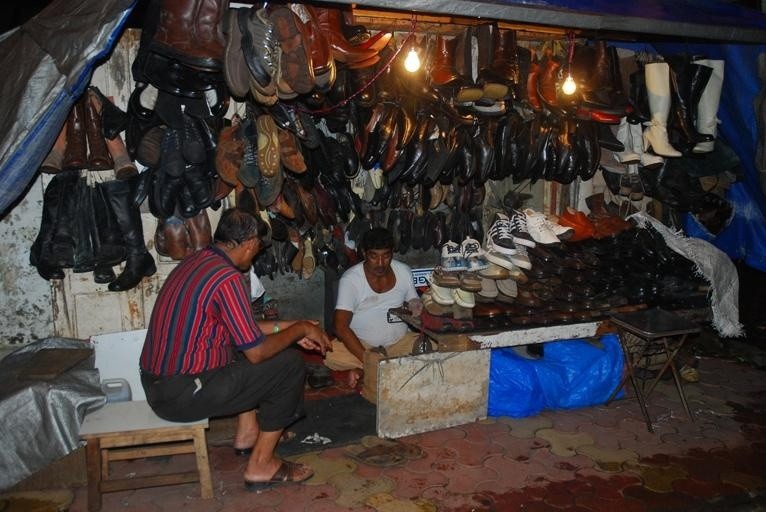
[139,207,331,484]
[321,226,437,389]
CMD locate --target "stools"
[602,304,702,434]
[77,399,212,511]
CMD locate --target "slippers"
[342,444,408,469]
[233,430,297,457]
[360,435,428,460]
[244,458,314,493]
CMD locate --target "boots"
[616,59,725,171]
[29,85,158,290]
[398,20,634,124]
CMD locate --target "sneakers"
[429,206,575,311]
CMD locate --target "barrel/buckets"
[101,378,132,401]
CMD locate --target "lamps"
[401,12,424,73]
[559,33,579,96]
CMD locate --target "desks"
[388,300,645,355]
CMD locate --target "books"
[14,347,94,380]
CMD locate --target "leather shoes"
[421,304,530,334]
[160,110,625,218]
[222,0,393,112]
[530,236,677,333]
[250,209,485,280]
[560,207,632,241]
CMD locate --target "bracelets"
[272,318,280,333]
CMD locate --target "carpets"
[250,391,376,459]
